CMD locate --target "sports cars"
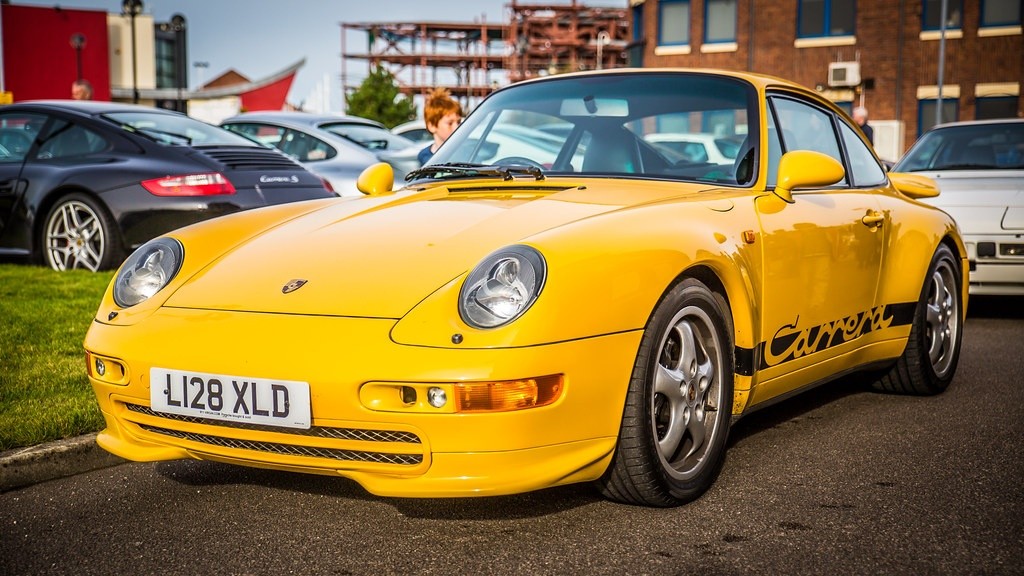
[80,67,972,511]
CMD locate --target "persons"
[72,82,106,154]
[852,106,874,147]
[417,86,482,164]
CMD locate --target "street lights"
[121,0,145,105]
[72,35,86,86]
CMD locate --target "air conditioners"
[827,61,861,87]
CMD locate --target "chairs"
[579,123,644,174]
[50,126,91,158]
[732,128,802,185]
[287,138,308,162]
[963,145,998,166]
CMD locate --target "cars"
[883,117,1024,298]
[217,111,434,202]
[395,118,745,190]
[0,100,342,274]
